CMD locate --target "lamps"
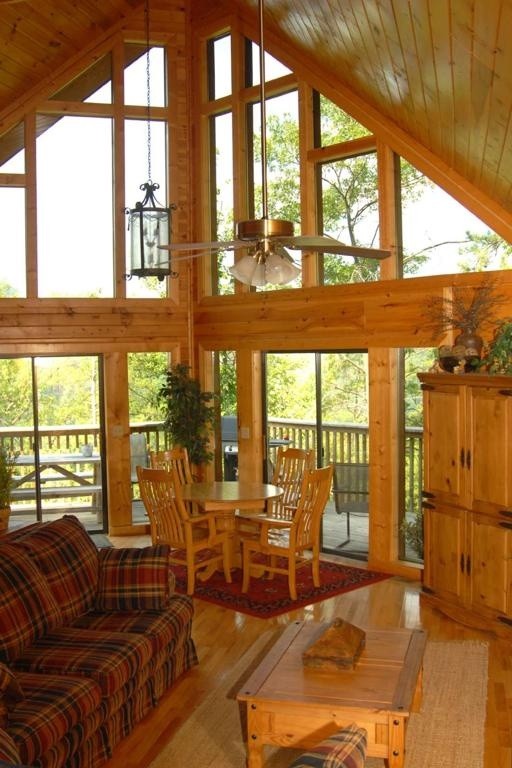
[121,0,180,281]
[228,251,301,287]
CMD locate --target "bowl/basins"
[438,353,480,375]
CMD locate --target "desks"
[6,451,103,523]
[165,481,285,585]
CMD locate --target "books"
[269,440,289,445]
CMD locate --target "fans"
[156,0,391,287]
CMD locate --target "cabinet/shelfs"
[417,371,512,627]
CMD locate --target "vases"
[453,326,485,359]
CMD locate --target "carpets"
[167,543,395,621]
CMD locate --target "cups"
[81,444,93,457]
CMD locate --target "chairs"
[332,462,369,549]
[136,464,233,596]
[242,464,332,601]
[130,434,150,503]
[239,448,316,561]
[150,448,239,562]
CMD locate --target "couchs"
[1,514,197,768]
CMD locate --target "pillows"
[0,661,25,702]
[96,543,170,613]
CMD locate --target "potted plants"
[0,445,20,532]
